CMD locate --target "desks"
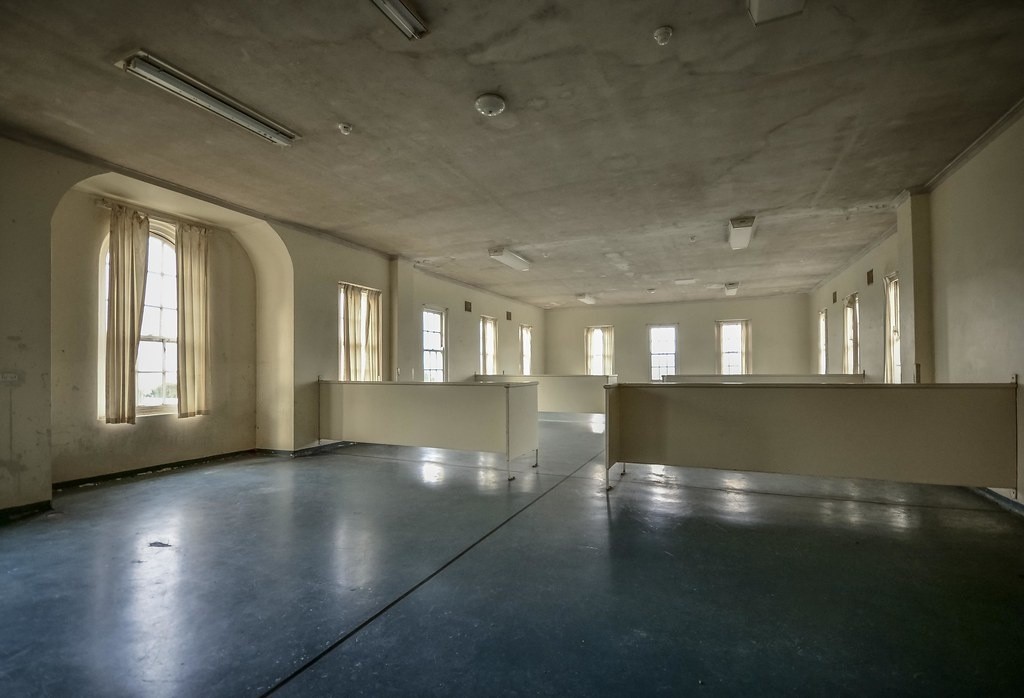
[661,371,868,384]
[600,378,1020,500]
[476,374,619,415]
[318,380,540,480]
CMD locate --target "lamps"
[578,294,596,306]
[113,50,302,145]
[725,283,739,296]
[489,244,532,278]
[365,0,431,40]
[730,218,756,251]
[748,0,806,27]
